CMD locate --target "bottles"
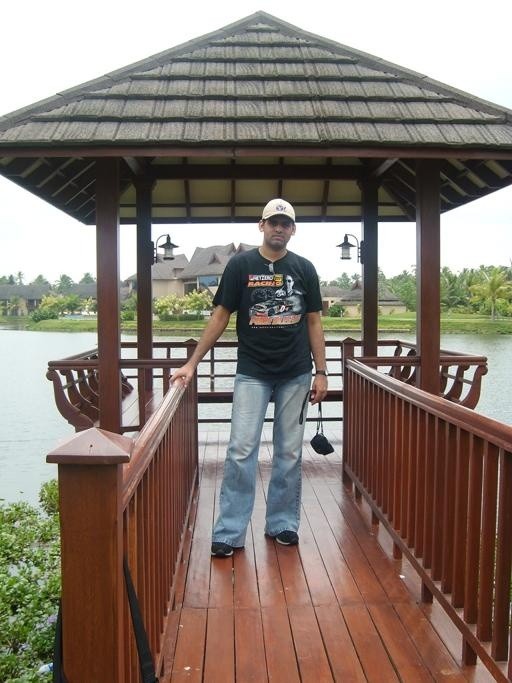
[262,198,296,223]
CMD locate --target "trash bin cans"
[316,370,329,376]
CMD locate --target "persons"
[170,198,328,557]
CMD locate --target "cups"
[285,280,291,283]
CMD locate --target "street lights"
[310,432,334,456]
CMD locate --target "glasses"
[337,234,364,264]
[150,234,178,265]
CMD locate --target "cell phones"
[311,433,334,455]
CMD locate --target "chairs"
[210,542,234,556]
[275,530,299,545]
